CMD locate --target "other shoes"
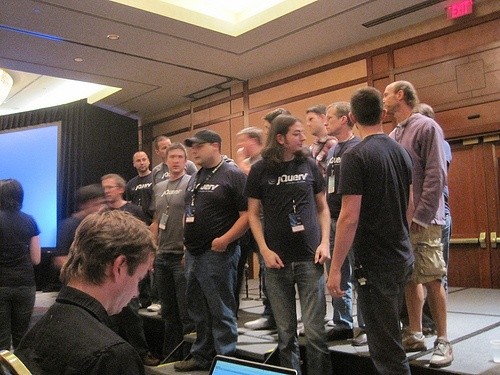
[173,358,212,371]
[326,328,354,341]
[351,331,368,346]
[147,304,160,311]
[244,317,276,330]
[142,352,160,366]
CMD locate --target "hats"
[184,129,221,148]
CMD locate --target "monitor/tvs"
[207,353,298,375]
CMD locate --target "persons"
[305,104,336,182]
[54,185,104,266]
[152,135,197,183]
[151,142,193,364]
[382,80,454,368]
[173,129,248,371]
[235,108,291,328]
[326,86,415,375]
[125,150,153,210]
[401,104,453,334]
[326,102,368,346]
[101,174,153,226]
[244,114,333,375]
[0,179,41,349]
[13,210,159,375]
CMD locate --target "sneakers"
[430,337,453,366]
[401,331,428,352]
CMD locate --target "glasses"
[102,185,124,190]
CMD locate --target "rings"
[334,290,336,292]
